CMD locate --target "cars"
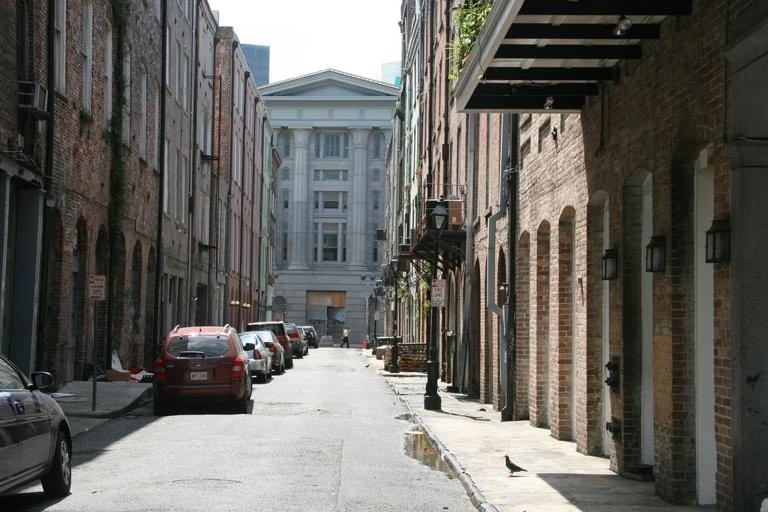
[0,353,71,494]
[154,321,319,414]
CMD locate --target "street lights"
[424,195,448,409]
[372,286,380,354]
[390,255,400,373]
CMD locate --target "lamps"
[600,245,618,281]
[645,235,666,274]
[704,215,732,263]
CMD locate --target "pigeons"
[365,364,369,368]
[504,455,528,477]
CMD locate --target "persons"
[340,327,350,348]
